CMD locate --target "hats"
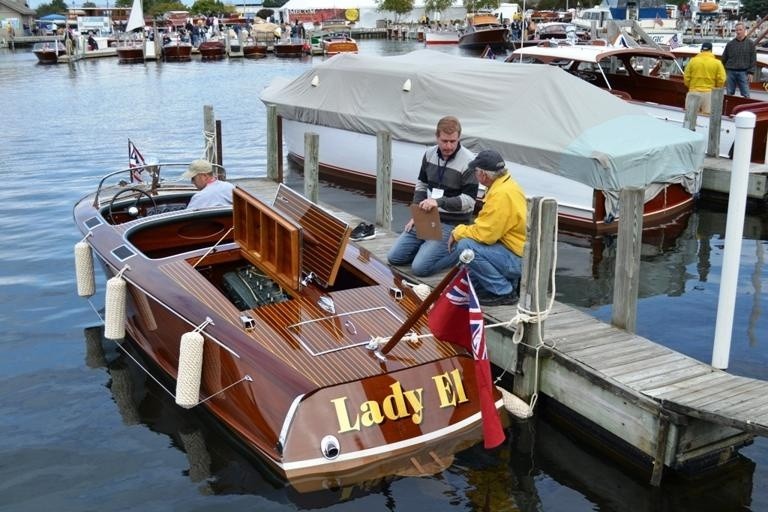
[183,160,212,178]
[468,150,505,170]
[701,42,712,50]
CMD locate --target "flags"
[425,262,509,450]
[669,34,681,51]
[127,139,146,184]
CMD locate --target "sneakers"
[478,290,518,306]
[349,223,376,241]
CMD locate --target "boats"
[425,0,768,102]
[72,138,511,495]
[31,25,359,65]
[257,49,704,239]
[503,43,768,166]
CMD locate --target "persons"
[387,118,481,277]
[720,22,758,99]
[180,158,236,211]
[683,42,727,114]
[446,147,530,307]
[6,15,308,49]
[500,17,533,43]
[463,426,520,512]
